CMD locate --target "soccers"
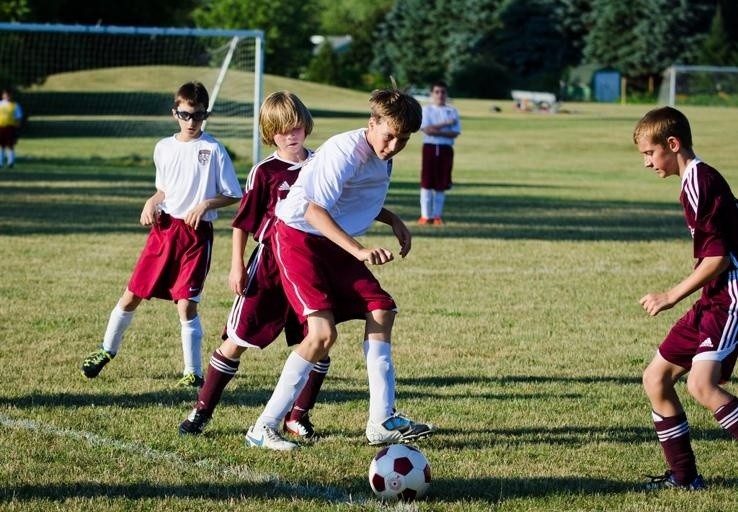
[368,444,431,500]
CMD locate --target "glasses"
[176,111,209,121]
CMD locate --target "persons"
[0,91,23,168]
[81,81,243,388]
[633,106,737,491]
[244,88,437,451]
[417,80,461,225]
[178,91,330,439]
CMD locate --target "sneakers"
[245,411,317,452]
[418,218,442,225]
[80,349,116,378]
[642,470,704,491]
[366,411,436,446]
[176,374,204,388]
[179,407,212,435]
